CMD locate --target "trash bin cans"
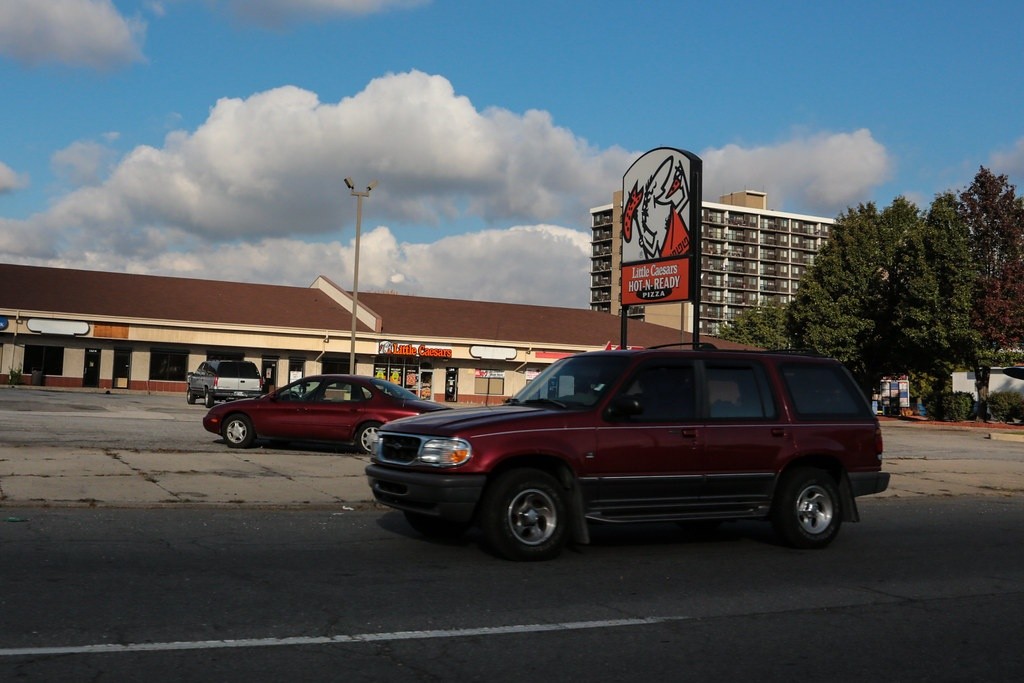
[31,370,42,386]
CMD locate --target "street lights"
[343,175,379,375]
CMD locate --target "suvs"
[186,359,264,408]
[363,342,892,559]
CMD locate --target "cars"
[202,374,454,457]
